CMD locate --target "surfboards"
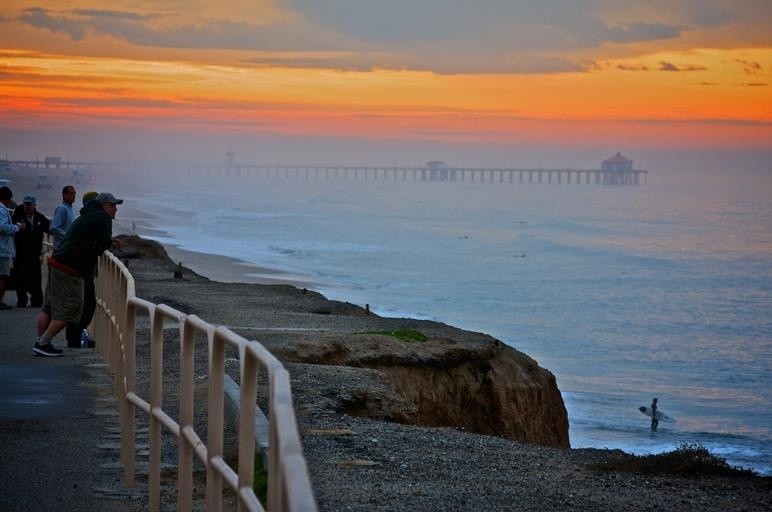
[638,407,678,424]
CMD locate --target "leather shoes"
[71,340,95,348]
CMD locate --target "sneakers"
[0,303,12,309]
[32,341,63,356]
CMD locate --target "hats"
[23,195,35,205]
[96,193,123,205]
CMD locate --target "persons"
[650,398,659,429]
[0,184,124,358]
